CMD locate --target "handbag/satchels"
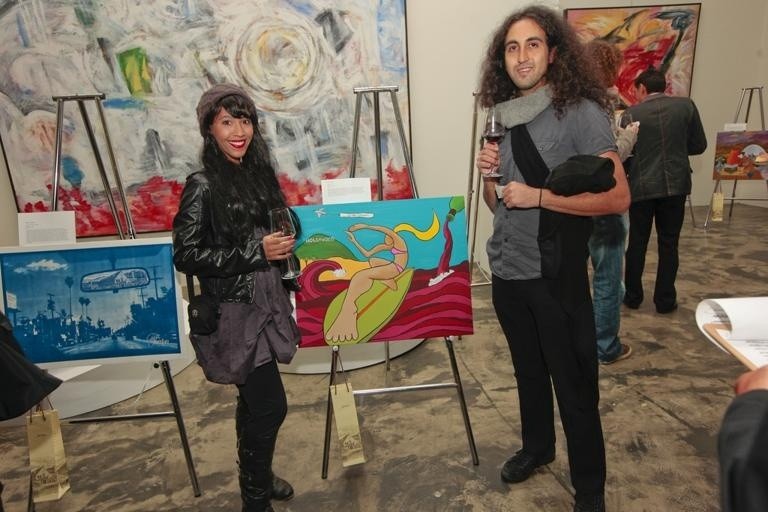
[26,408,70,503]
[188,295,219,333]
[328,383,366,468]
[713,191,723,224]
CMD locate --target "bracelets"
[537,188,545,207]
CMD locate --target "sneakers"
[599,344,632,365]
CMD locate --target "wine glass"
[482,109,505,179]
[269,207,302,280]
[619,114,634,158]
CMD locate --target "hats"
[196,82,251,124]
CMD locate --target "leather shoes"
[574,490,605,512]
[500,449,555,483]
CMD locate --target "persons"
[617,70,707,314]
[574,39,638,364]
[475,5,632,510]
[172,83,304,512]
[717,365,768,511]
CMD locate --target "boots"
[236,400,293,511]
